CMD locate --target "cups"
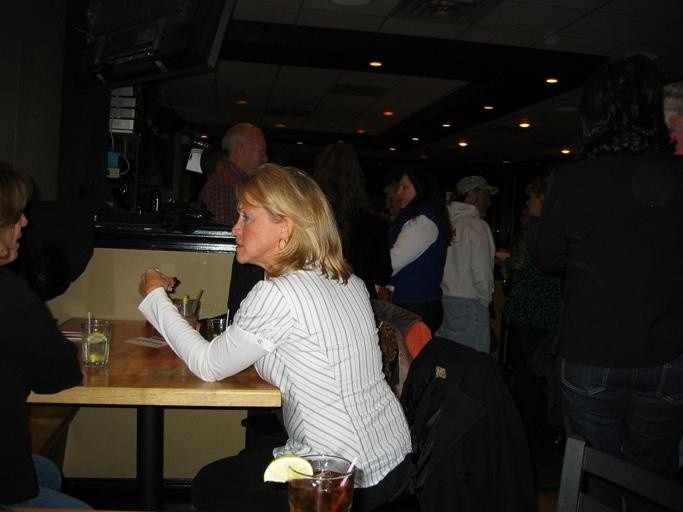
[176,298,198,330]
[80,319,110,366]
[205,318,232,341]
[285,455,356,512]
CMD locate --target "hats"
[455,175,499,196]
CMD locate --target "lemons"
[264,456,314,483]
[87,331,108,345]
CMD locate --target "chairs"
[27,299,683,512]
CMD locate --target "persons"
[661,78,681,159]
[208,124,233,168]
[531,55,681,510]
[200,122,267,224]
[316,138,394,300]
[0,162,104,507]
[138,161,411,512]
[272,133,311,170]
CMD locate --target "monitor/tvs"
[183,144,210,177]
[76,0,237,93]
